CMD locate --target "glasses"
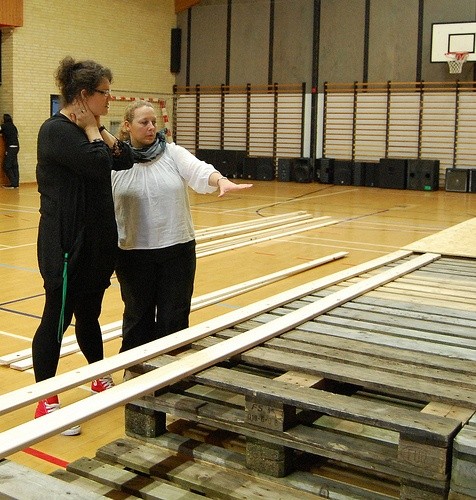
[93,89,111,97]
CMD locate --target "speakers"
[444,168,476,192]
[193,149,439,193]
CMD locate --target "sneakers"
[91,374,116,394]
[35,395,81,435]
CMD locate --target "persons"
[32,56,135,439]
[0,114,19,189]
[111,101,254,385]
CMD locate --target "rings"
[82,110,85,113]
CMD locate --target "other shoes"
[2,184,19,190]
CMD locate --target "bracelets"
[99,126,105,133]
[217,176,227,187]
